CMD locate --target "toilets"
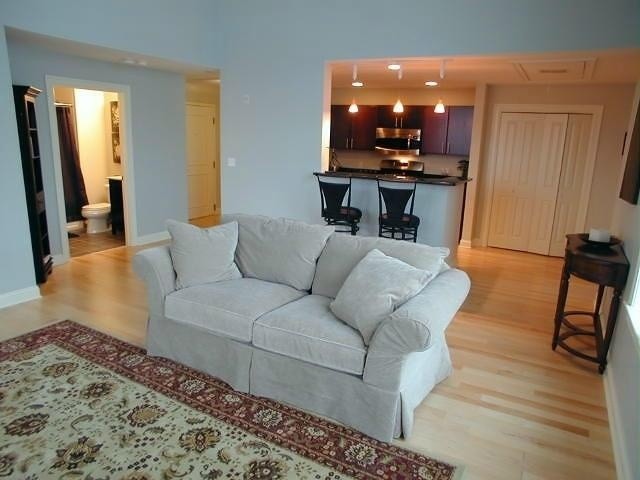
[81,183,112,234]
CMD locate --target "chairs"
[376,177,420,243]
[313,172,362,236]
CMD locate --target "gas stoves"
[378,173,417,182]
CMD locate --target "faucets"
[329,152,341,173]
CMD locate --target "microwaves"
[375,127,420,156]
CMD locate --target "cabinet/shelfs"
[330,105,379,151]
[420,106,474,156]
[12,85,53,283]
[378,105,420,128]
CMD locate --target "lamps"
[349,98,445,113]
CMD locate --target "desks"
[552,233,630,375]
[105,176,124,234]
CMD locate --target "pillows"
[312,233,450,299]
[329,249,433,345]
[234,216,335,291]
[167,220,243,290]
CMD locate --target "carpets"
[0,319,467,479]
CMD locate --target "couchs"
[131,215,471,445]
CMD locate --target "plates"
[578,234,621,248]
